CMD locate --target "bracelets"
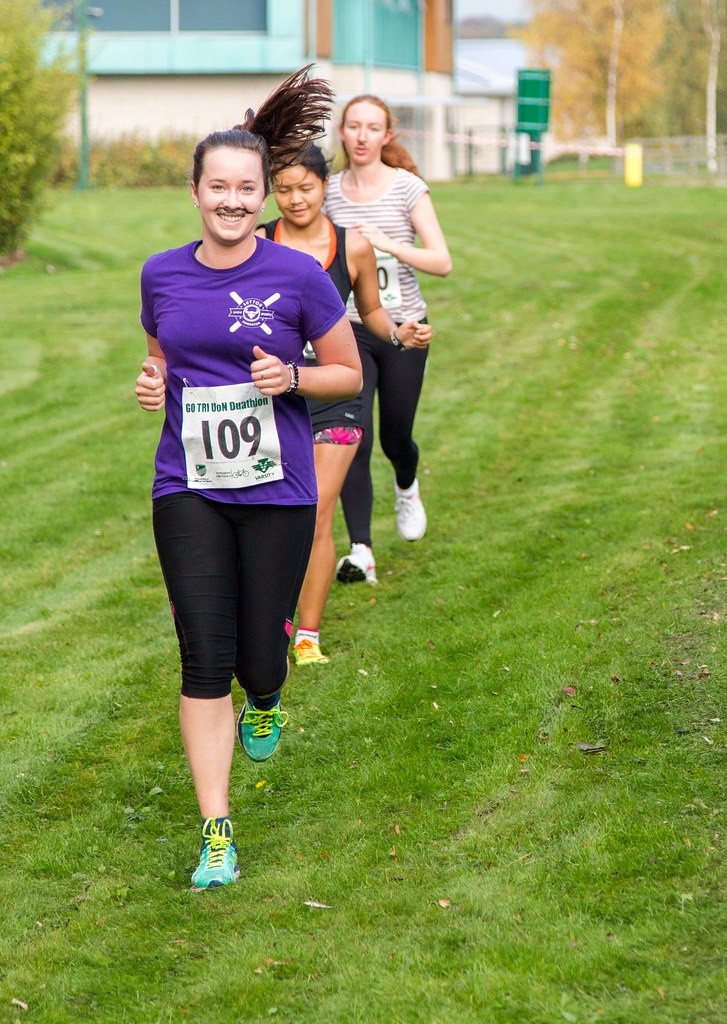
[391,331,408,352]
[285,360,299,395]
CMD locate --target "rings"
[260,370,264,380]
[151,364,157,376]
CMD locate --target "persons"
[255,139,433,666]
[135,61,364,890]
[321,96,453,585]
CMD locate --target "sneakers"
[293,639,329,664]
[392,476,428,542]
[236,691,289,761]
[335,543,378,585]
[190,817,240,894]
